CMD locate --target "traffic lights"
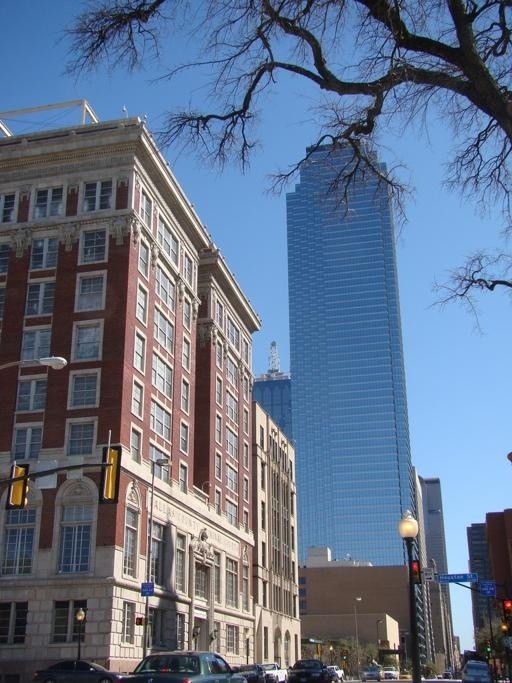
[486,646,491,653]
[502,600,512,611]
[412,561,421,584]
[135,617,141,626]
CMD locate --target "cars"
[118,650,248,683]
[233,659,345,683]
[35,660,119,682]
[360,665,452,682]
[462,660,493,683]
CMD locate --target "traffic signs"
[439,573,479,581]
[421,566,433,582]
[480,581,497,597]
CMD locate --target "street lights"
[329,645,333,665]
[398,508,423,683]
[376,618,384,650]
[354,597,364,679]
[77,607,85,662]
[1,355,67,371]
[143,457,174,661]
